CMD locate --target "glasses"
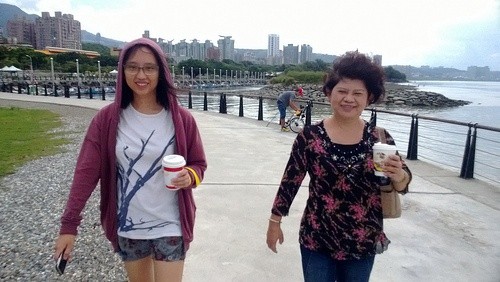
[123,63,159,76]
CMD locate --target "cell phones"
[56,248,68,276]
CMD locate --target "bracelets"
[268,218,282,224]
[391,169,406,184]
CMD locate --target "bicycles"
[285,99,314,134]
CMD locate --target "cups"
[162,154,186,190]
[372,143,399,177]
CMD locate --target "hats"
[298,87,303,96]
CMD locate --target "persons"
[54,38,207,282]
[267,49,412,282]
[277,87,303,132]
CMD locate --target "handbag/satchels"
[375,126,402,219]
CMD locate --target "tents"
[109,70,119,78]
[0,65,23,77]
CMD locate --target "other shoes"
[281,126,289,132]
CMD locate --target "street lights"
[76,59,79,80]
[50,57,54,83]
[97,61,101,80]
[26,55,33,82]
